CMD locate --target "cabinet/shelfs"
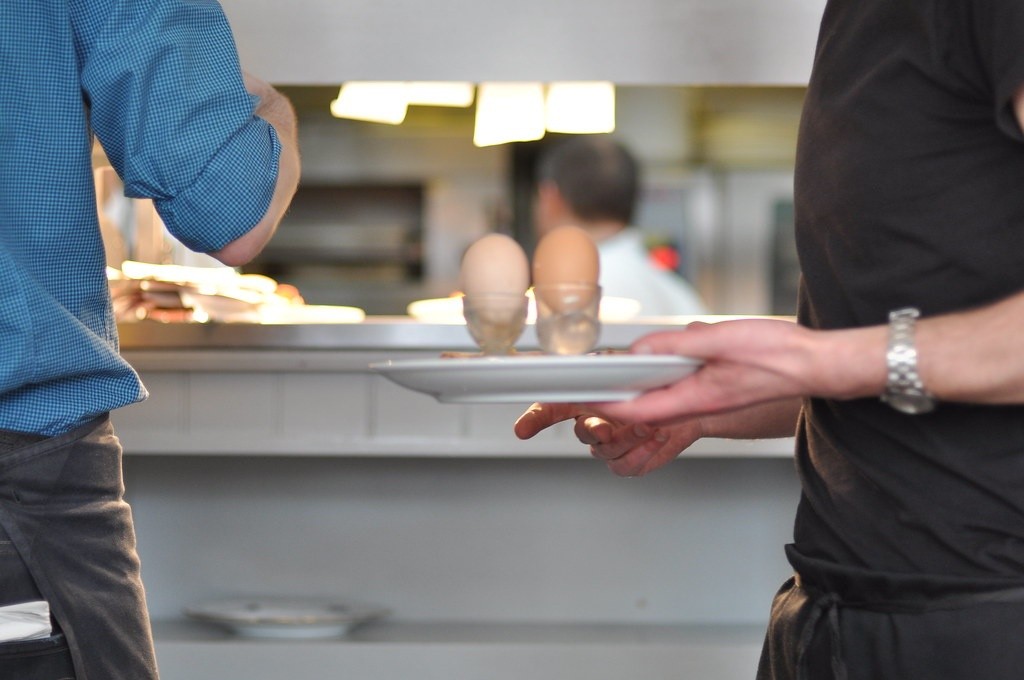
[109,345,800,680]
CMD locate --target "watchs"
[879,306,939,417]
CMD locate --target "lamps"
[472,82,616,148]
[330,81,476,127]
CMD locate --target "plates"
[369,353,709,406]
[180,600,393,643]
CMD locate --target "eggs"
[532,227,599,311]
[460,232,529,322]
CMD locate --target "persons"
[513,0,1024,680]
[0,0,301,680]
[530,135,708,316]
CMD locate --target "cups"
[462,293,530,357]
[532,284,603,353]
